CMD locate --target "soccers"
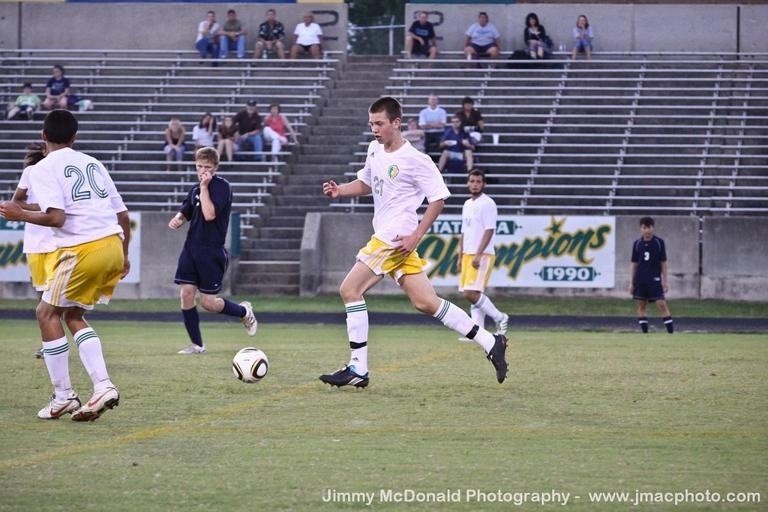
[231,347,268,383]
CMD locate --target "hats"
[247,99,256,105]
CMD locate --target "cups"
[492,133,499,144]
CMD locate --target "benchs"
[1,45,345,242]
[327,48,767,212]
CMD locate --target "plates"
[443,140,456,145]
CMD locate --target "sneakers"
[177,343,206,355]
[457,313,509,383]
[239,300,258,336]
[318,363,369,389]
[70,385,120,422]
[37,389,81,419]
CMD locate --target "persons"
[228,99,265,161]
[405,10,438,61]
[41,62,71,110]
[193,9,220,67]
[523,12,547,61]
[248,8,286,69]
[454,167,511,343]
[260,101,299,161]
[417,91,449,148]
[288,11,327,69]
[165,144,259,355]
[190,112,216,163]
[435,114,476,173]
[462,11,503,60]
[570,14,594,60]
[0,106,133,425]
[215,116,234,162]
[217,10,248,60]
[628,216,675,333]
[4,81,41,123]
[453,95,485,149]
[318,95,509,392]
[399,116,426,155]
[10,137,49,358]
[159,116,185,172]
